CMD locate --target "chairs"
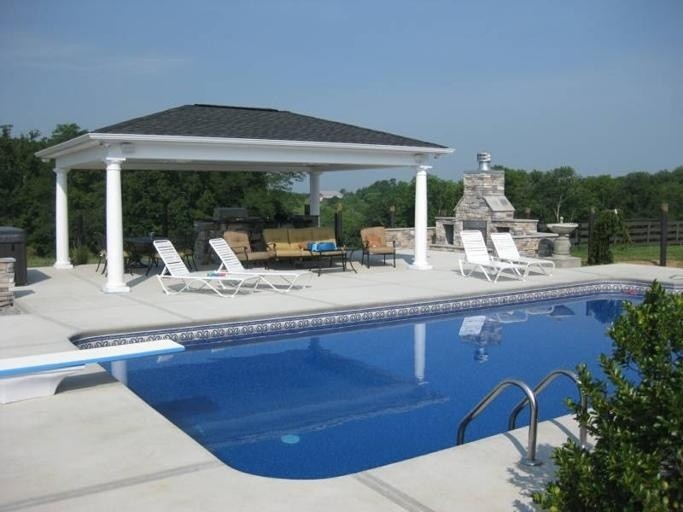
[360,226,398,270]
[458,229,556,285]
[86,218,314,296]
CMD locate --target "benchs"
[261,225,346,270]
